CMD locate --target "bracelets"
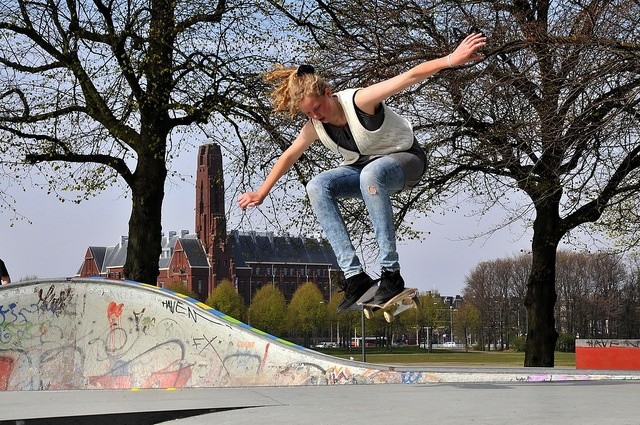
[448,54,453,68]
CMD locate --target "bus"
[351,336,388,347]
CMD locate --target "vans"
[443,342,456,348]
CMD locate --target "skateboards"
[356,285,422,323]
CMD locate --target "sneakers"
[373,266,404,301]
[336,272,375,315]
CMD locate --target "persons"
[0,259,11,285]
[237,33,487,315]
